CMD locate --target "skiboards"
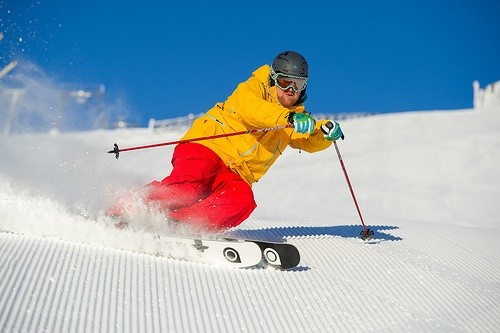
[70,216,301,270]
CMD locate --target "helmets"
[272,50,308,79]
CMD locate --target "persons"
[104,50,345,234]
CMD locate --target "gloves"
[289,112,315,134]
[320,119,344,140]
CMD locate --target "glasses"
[269,64,308,92]
